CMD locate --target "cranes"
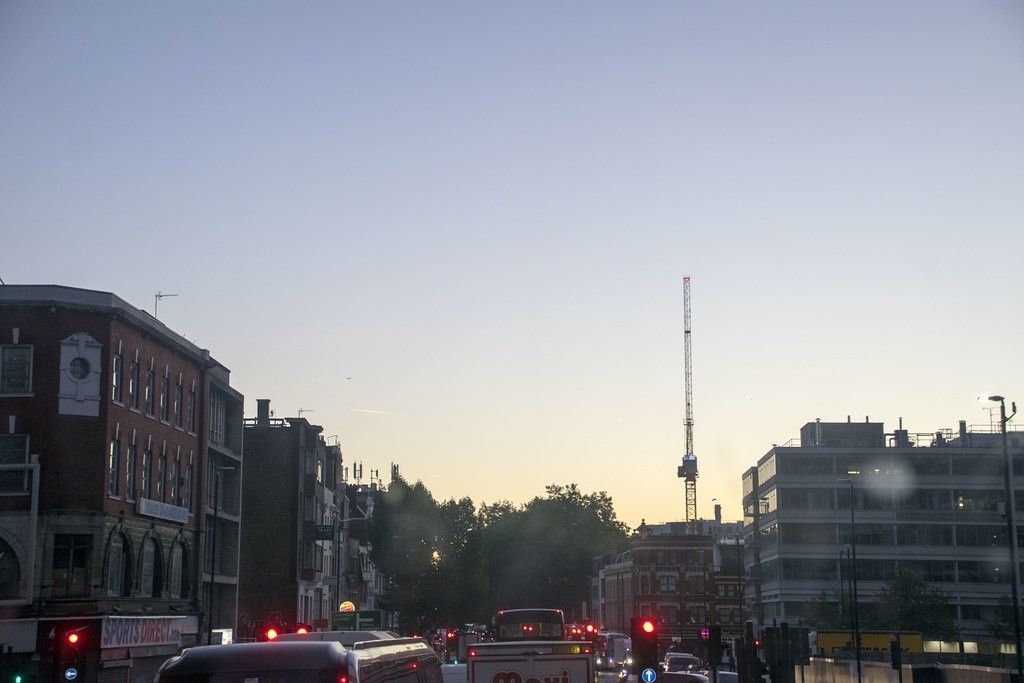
[677,275,700,523]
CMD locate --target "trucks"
[465,637,595,683]
[456,631,481,664]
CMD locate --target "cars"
[659,651,738,683]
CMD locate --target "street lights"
[728,533,743,637]
[988,395,1024,683]
[843,543,854,651]
[336,517,351,611]
[207,466,236,646]
[695,549,707,646]
[836,478,861,683]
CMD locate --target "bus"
[152,628,446,683]
[568,624,633,670]
[496,608,566,641]
